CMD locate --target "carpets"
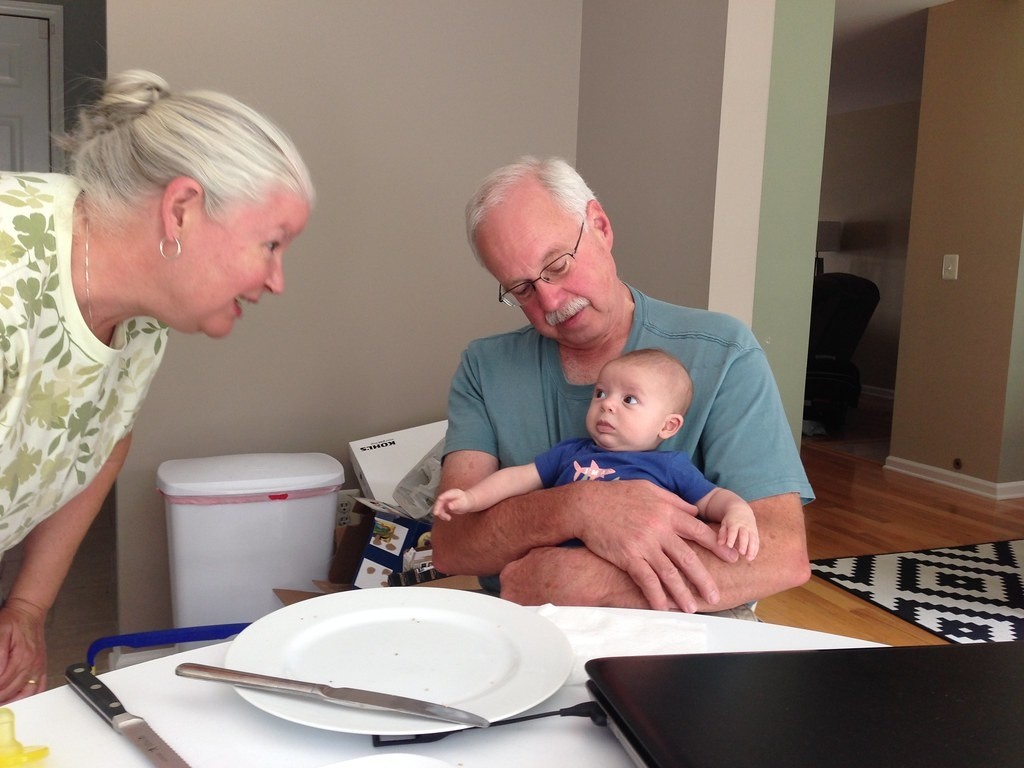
[807,536,1024,644]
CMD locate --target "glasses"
[498,220,586,308]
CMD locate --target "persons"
[0,68,317,718]
[429,154,820,624]
[429,350,767,624]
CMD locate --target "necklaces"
[85,217,97,338]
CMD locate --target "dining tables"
[0,598,1023,767]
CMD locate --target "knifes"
[175,662,492,728]
[63,662,194,768]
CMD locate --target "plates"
[222,586,573,736]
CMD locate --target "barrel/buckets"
[158,452,345,651]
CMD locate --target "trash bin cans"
[157,452,347,651]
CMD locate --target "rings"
[26,679,41,685]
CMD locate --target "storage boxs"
[156,451,345,654]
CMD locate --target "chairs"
[802,272,880,439]
